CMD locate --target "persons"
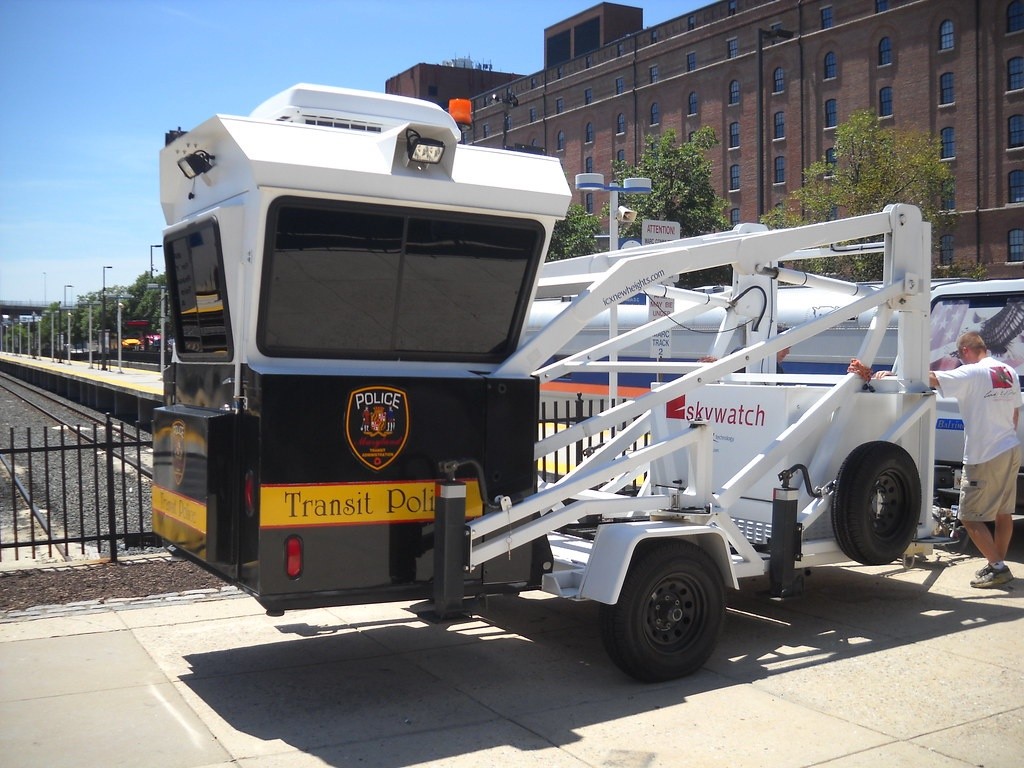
[873,331,1021,588]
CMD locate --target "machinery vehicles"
[156,82,962,680]
[517,277,964,494]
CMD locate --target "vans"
[890,276,1024,522]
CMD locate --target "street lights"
[0,244,169,382]
[573,171,653,410]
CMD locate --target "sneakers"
[971,564,1015,589]
